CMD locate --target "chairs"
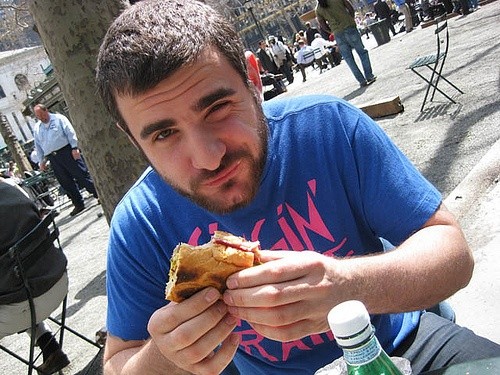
[404,12,464,112]
[302,48,331,74]
[413,93,466,123]
[0,209,113,375]
[23,172,89,212]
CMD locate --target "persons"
[392,0,413,33]
[258,21,314,91]
[329,34,342,68]
[0,178,70,375]
[314,0,378,88]
[364,13,373,39]
[0,158,24,178]
[33,103,98,216]
[470,0,481,11]
[311,33,336,69]
[374,0,398,36]
[94,0,500,375]
[454,0,470,17]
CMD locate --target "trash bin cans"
[368,17,391,46]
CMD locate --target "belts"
[44,144,71,161]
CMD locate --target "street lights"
[243,1,267,41]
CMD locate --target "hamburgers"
[165,231,261,307]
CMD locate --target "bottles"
[327,300,402,375]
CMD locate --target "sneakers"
[36,351,70,375]
[70,206,85,216]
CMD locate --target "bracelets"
[72,147,77,150]
